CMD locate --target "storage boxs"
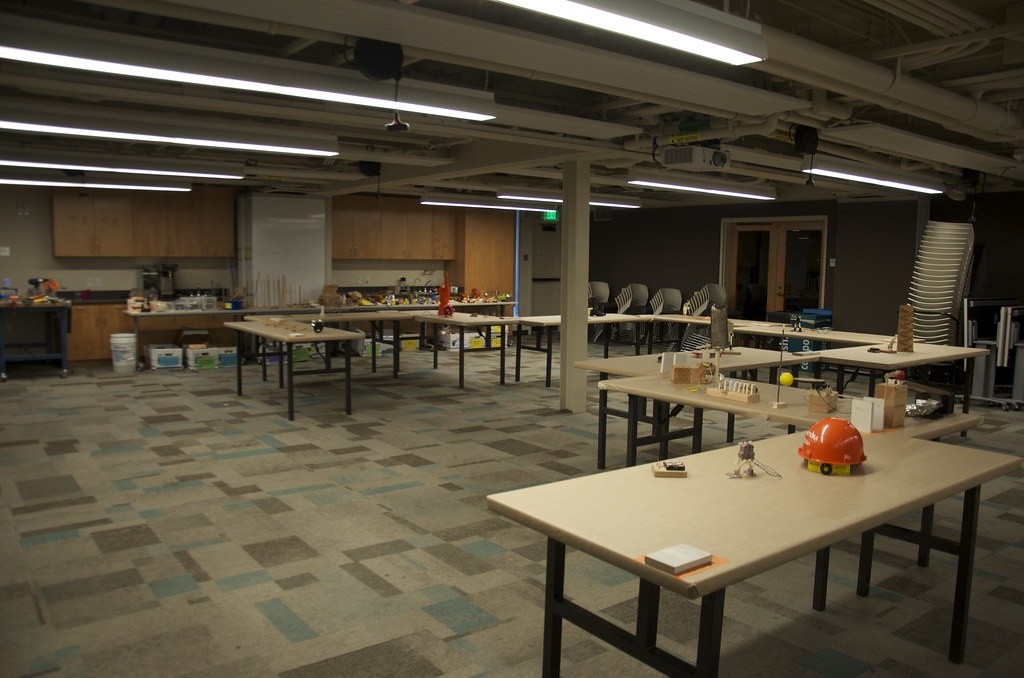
[882,406,907,427]
[142,324,511,371]
[809,391,840,414]
[875,384,904,405]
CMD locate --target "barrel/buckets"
[109,333,138,374]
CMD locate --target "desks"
[819,343,990,444]
[595,378,981,472]
[733,325,928,392]
[243,311,409,390]
[485,431,1024,678]
[516,310,651,388]
[412,313,519,388]
[575,345,819,468]
[223,323,366,421]
[646,315,792,381]
[0,300,71,381]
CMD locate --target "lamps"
[0,0,945,212]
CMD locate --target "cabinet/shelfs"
[433,200,457,260]
[441,211,518,333]
[133,190,202,258]
[333,193,381,260]
[201,187,240,258]
[192,302,234,329]
[379,198,434,260]
[53,193,132,259]
[69,305,134,360]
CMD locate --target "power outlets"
[95,278,102,286]
[88,278,93,284]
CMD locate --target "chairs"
[899,221,977,388]
[588,280,726,351]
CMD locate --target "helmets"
[799,417,866,465]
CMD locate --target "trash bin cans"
[788,308,832,373]
[764,312,787,351]
